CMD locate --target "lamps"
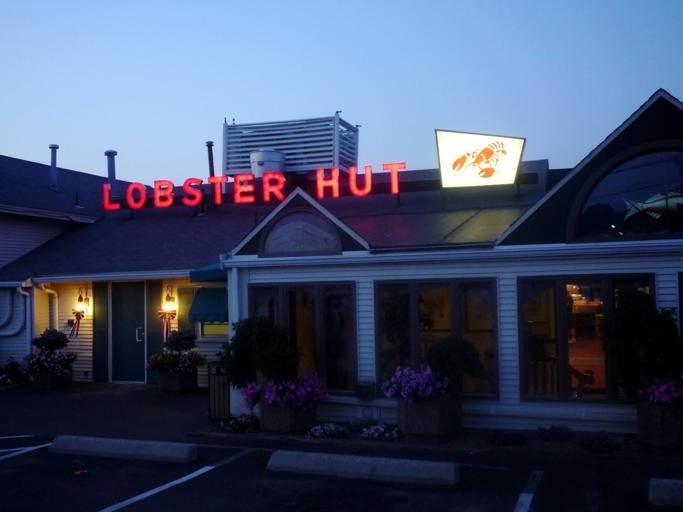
[163,286,175,311]
[296,326,315,362]
[75,287,89,313]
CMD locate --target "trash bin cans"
[209,361,231,422]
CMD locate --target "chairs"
[490,353,593,403]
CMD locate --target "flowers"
[148,352,174,369]
[241,372,328,414]
[179,350,198,368]
[635,379,683,405]
[379,366,450,402]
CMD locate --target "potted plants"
[158,330,199,391]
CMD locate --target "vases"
[157,363,174,370]
[636,403,683,449]
[262,405,317,431]
[398,397,463,436]
